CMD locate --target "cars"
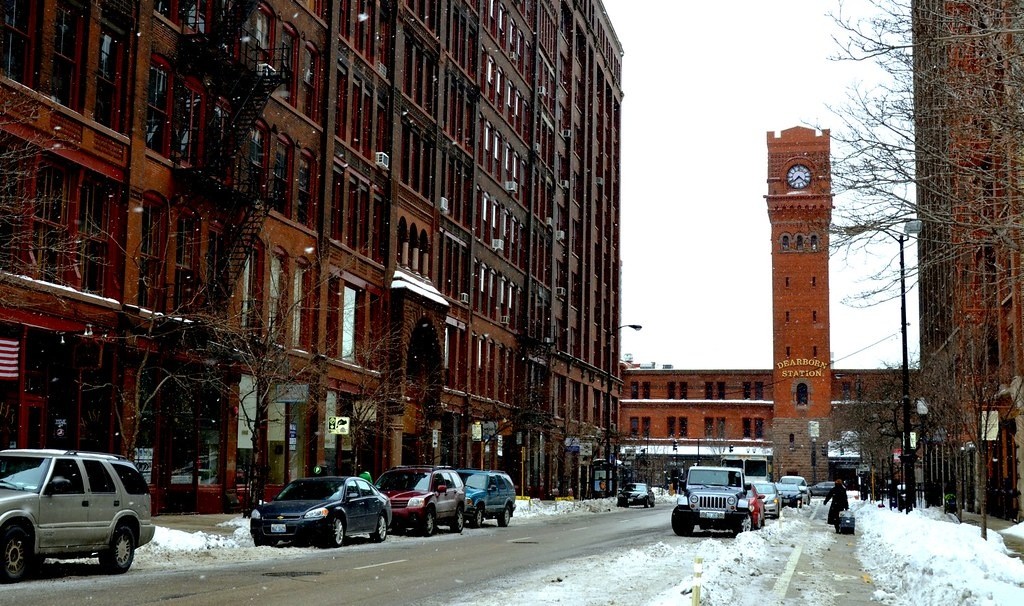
[753,482,780,518]
[250,476,392,549]
[781,475,812,505]
[774,483,803,507]
[744,483,766,530]
[617,482,654,508]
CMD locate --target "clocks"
[787,164,812,189]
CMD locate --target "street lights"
[898,219,923,511]
[854,375,865,468]
[915,394,930,510]
[606,324,643,495]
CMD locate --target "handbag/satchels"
[839,510,854,517]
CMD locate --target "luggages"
[840,508,856,537]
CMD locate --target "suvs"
[451,467,518,528]
[670,465,754,539]
[1,444,155,583]
[371,464,467,535]
[809,480,848,495]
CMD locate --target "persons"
[823,478,850,534]
[360,467,374,485]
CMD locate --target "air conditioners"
[492,239,505,252]
[555,287,566,296]
[375,152,391,171]
[560,179,570,189]
[556,231,565,241]
[537,86,547,97]
[561,129,573,138]
[546,217,555,229]
[460,293,470,304]
[544,337,551,344]
[440,196,448,211]
[535,143,542,154]
[257,63,278,76]
[505,181,520,193]
[500,316,512,325]
[596,177,604,186]
[510,52,517,61]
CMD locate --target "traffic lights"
[840,448,844,455]
